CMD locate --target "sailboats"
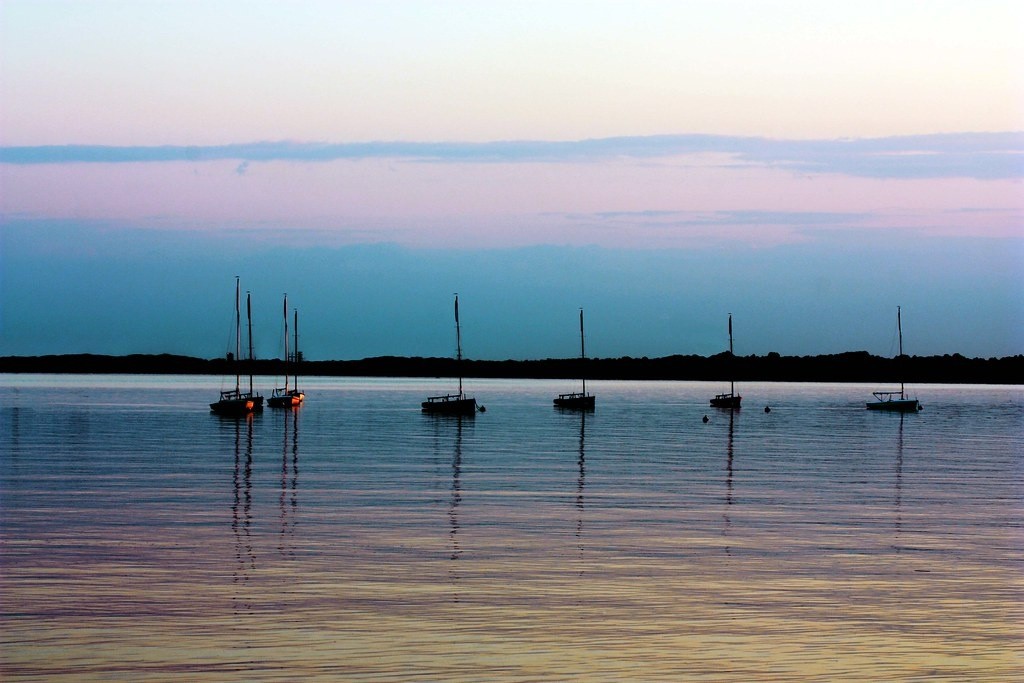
[707,311,745,409]
[268,293,305,407]
[864,304,927,416]
[208,275,264,418]
[422,291,487,418]
[552,308,595,415]
